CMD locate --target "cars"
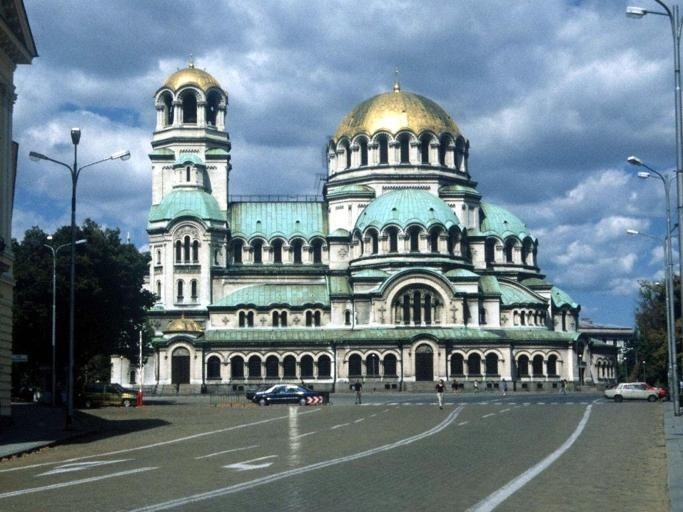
[605,383,658,402]
[246,383,319,406]
[78,384,137,409]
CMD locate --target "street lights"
[624,357,628,382]
[626,156,680,416]
[625,0,683,345]
[579,354,582,391]
[29,128,130,429]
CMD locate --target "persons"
[473,379,479,396]
[559,379,569,395]
[353,379,362,404]
[501,378,507,396]
[435,379,446,409]
